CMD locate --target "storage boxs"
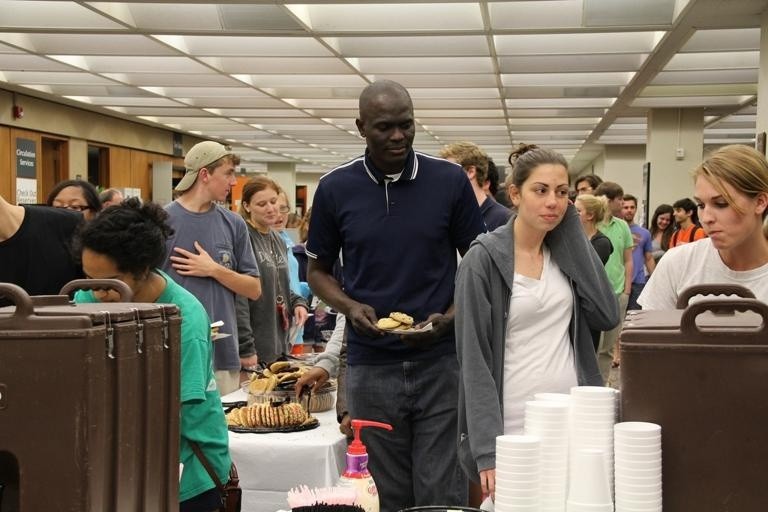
[618,282,768,512]
[0,276,179,512]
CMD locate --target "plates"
[382,322,434,335]
[210,332,232,340]
[227,418,321,434]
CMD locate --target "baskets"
[247,393,335,412]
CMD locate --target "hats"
[175,140,234,192]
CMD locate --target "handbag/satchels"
[219,461,246,512]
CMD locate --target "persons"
[440,141,706,386]
[74,196,232,511]
[453,141,621,512]
[636,145,768,309]
[1,140,352,442]
[305,79,487,511]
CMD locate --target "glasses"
[279,205,291,213]
[55,205,90,212]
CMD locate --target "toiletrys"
[340,419,393,512]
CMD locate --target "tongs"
[223,352,311,418]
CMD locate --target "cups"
[495,387,662,512]
